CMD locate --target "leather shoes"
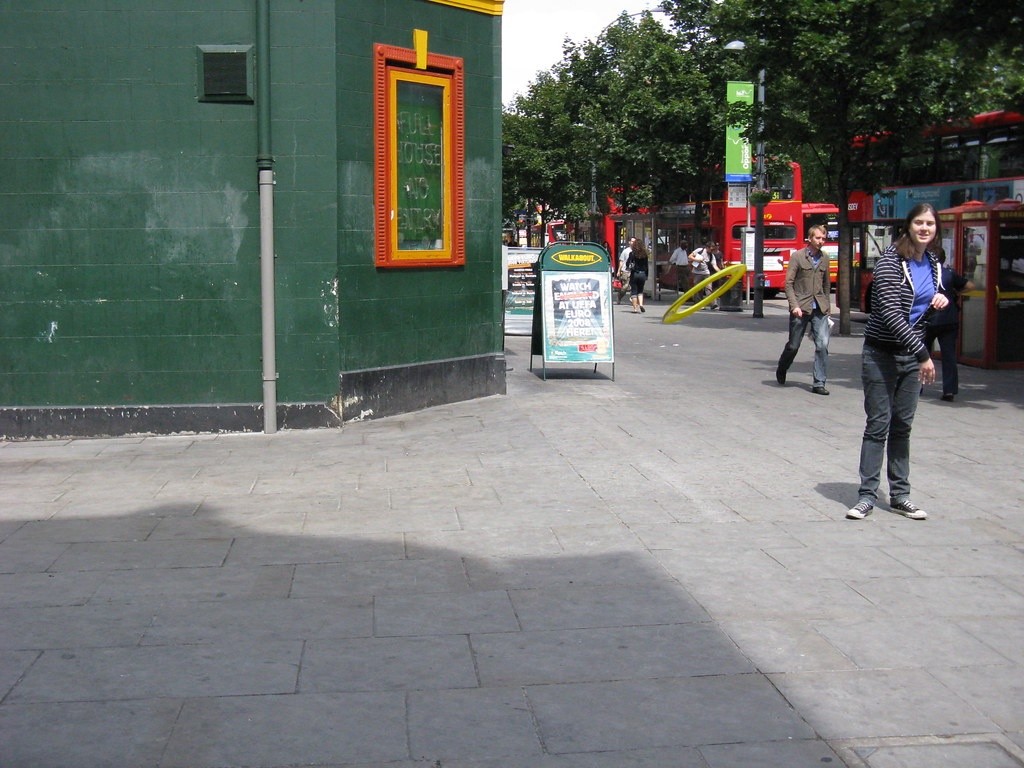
[776,363,787,384]
[812,385,830,395]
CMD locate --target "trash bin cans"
[718,275,744,313]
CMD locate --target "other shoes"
[640,305,646,313]
[630,310,639,313]
[940,394,954,402]
[617,295,621,304]
[711,304,719,310]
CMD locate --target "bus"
[530,109,1024,315]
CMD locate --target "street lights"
[723,39,765,319]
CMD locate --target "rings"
[924,373,927,376]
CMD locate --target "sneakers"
[889,497,928,519]
[846,502,873,518]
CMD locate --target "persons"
[556,236,563,241]
[688,241,721,310]
[603,242,611,257]
[626,239,648,313]
[507,236,518,247]
[616,238,636,305]
[776,224,832,395]
[920,248,974,401]
[670,240,688,293]
[708,240,724,290]
[846,203,949,519]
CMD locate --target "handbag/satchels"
[612,274,622,288]
[807,318,835,345]
[625,260,636,271]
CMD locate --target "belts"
[678,265,689,267]
